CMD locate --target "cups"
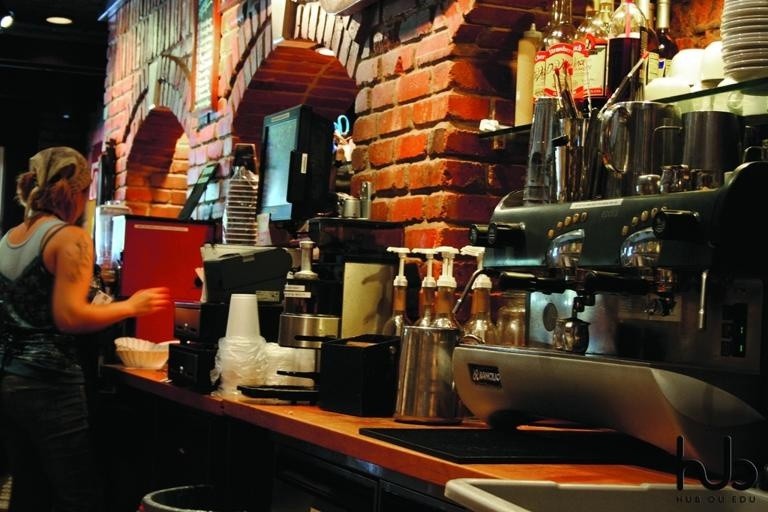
[599,100,678,198]
[226,142,260,246]
[523,95,601,206]
[391,325,484,428]
[220,291,267,394]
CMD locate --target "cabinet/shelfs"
[227,417,470,512]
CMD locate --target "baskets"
[113,336,169,369]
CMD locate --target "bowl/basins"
[113,337,167,370]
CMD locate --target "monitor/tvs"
[254,103,330,249]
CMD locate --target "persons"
[0,146,169,511]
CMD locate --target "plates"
[718,1,767,83]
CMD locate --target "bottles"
[510,0,677,128]
